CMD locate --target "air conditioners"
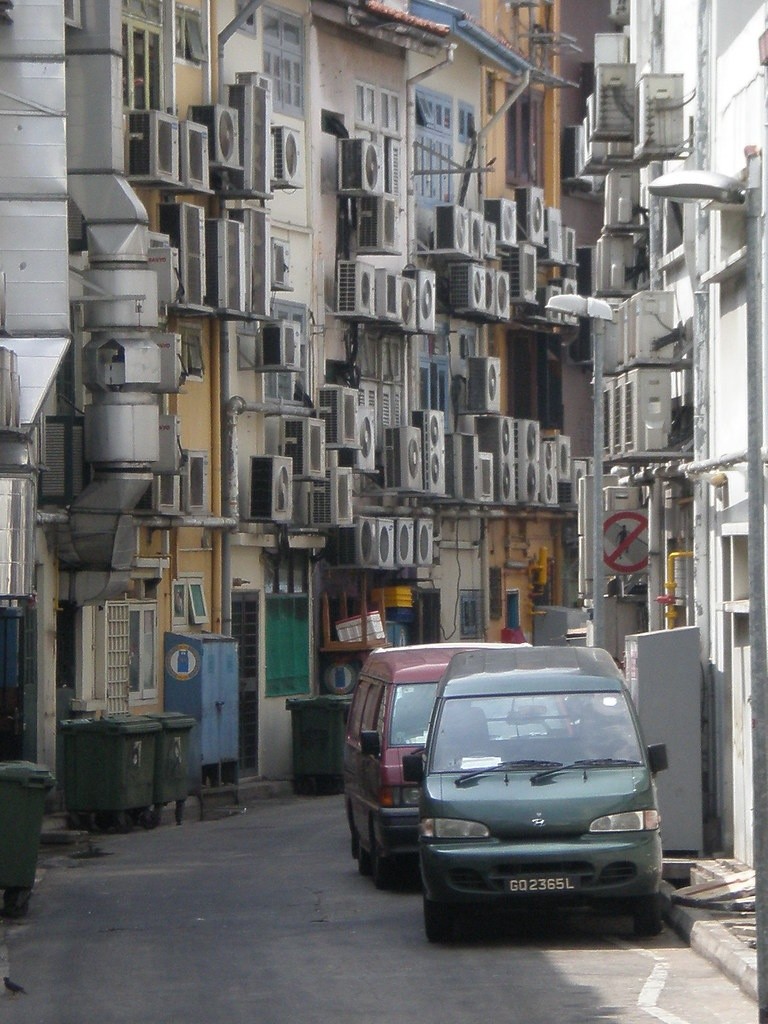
[125,0,689,573]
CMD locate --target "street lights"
[547,293,614,651]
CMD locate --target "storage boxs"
[335,585,413,672]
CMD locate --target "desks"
[320,574,394,652]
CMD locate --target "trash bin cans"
[57,710,207,834]
[284,693,356,796]
[0,760,57,917]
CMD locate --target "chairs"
[458,707,495,756]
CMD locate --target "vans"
[344,642,533,893]
[402,646,671,944]
